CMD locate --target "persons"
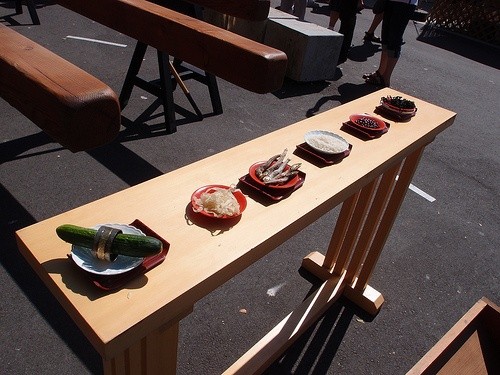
[277,0,417,93]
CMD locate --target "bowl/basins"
[381,97,416,119]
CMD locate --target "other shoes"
[306,2,319,8]
[362,70,390,87]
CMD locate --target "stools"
[203,6,344,81]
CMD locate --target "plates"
[296,130,353,163]
[239,159,306,200]
[66,219,171,291]
[342,114,391,139]
[191,185,247,218]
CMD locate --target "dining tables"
[18,85,456,375]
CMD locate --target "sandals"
[363,31,382,43]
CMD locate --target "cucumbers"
[56,225,162,257]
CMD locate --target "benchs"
[0,23,122,153]
[53,0,287,134]
